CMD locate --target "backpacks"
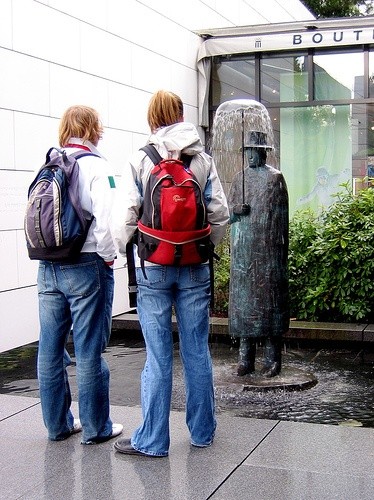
[23,146,105,260]
[136,144,221,266]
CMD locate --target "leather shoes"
[113,438,164,458]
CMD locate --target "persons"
[228,131,290,378]
[113,89,230,456]
[24,106,125,447]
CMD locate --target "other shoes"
[110,423,123,438]
[73,418,83,433]
